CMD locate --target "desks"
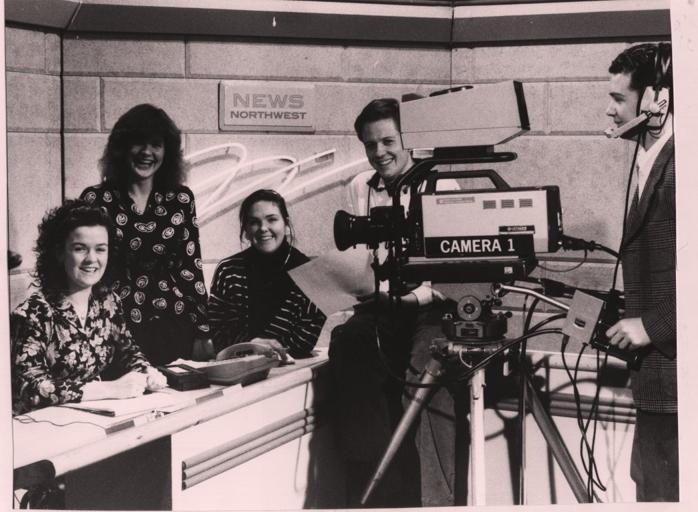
[13,346,335,510]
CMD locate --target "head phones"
[635,42,672,129]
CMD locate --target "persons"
[205,185,325,364]
[9,196,167,412]
[331,95,464,306]
[596,41,679,503]
[75,98,211,367]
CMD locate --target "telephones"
[196,342,280,387]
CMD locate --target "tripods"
[356,339,597,503]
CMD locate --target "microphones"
[603,114,648,139]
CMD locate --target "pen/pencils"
[74,407,115,417]
